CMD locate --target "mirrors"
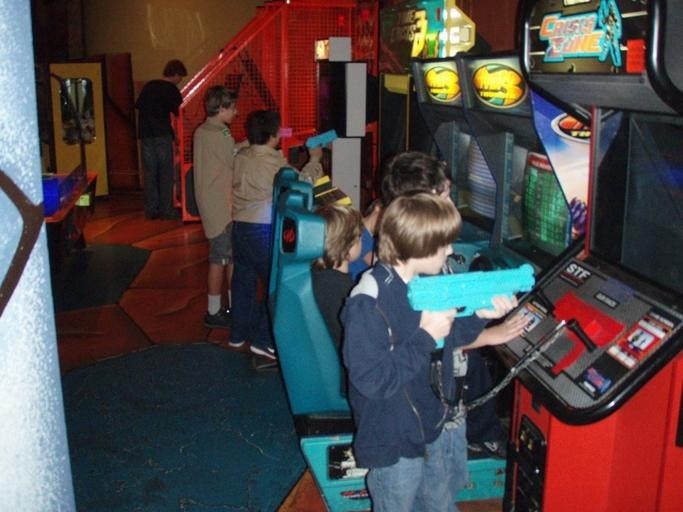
[58,79,96,145]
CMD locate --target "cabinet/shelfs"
[48,65,111,199]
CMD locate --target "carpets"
[60,339,307,511]
[50,244,151,312]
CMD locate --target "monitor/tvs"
[463,133,496,219]
[618,113,683,298]
[519,153,570,257]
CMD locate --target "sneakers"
[144,208,182,220]
[251,344,278,360]
[467,432,509,459]
[204,307,232,329]
[229,337,246,348]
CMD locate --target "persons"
[227,109,323,361]
[135,59,188,221]
[304,204,365,396]
[192,85,250,329]
[336,191,519,512]
[380,151,533,459]
[348,195,384,282]
